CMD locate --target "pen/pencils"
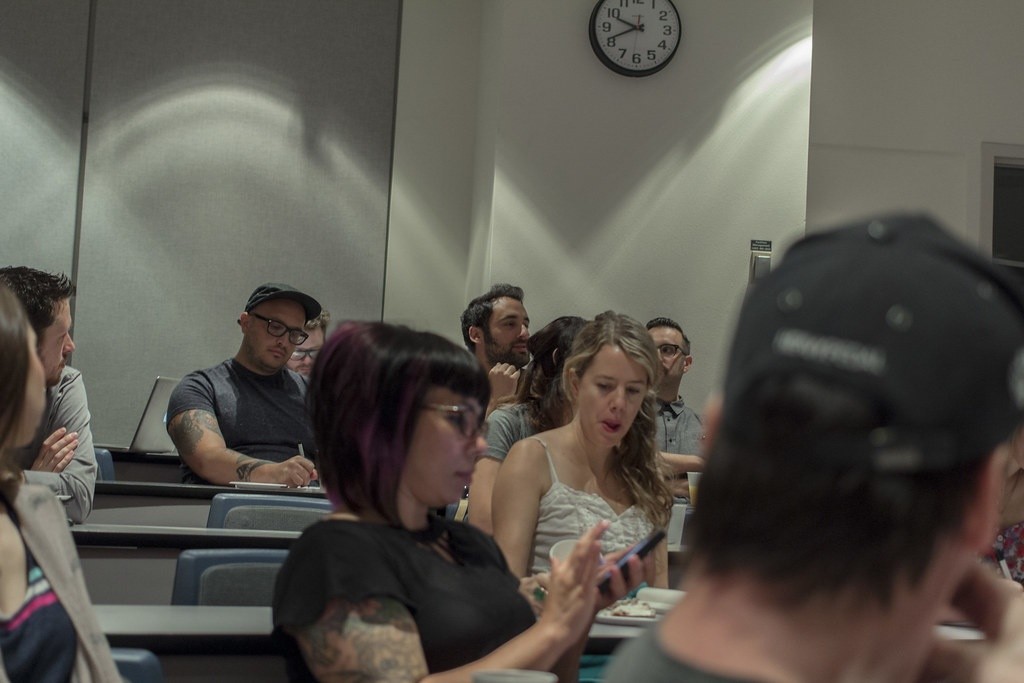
[298,440,311,487]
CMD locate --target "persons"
[0,264,99,525]
[644,318,712,502]
[0,277,126,683]
[286,310,328,376]
[600,207,1024,683]
[462,283,532,412]
[467,314,595,534]
[492,309,679,616]
[166,283,327,489]
[272,318,647,683]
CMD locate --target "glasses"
[248,314,309,345]
[655,342,690,358]
[422,401,489,440]
[290,346,320,361]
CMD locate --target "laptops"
[91,376,183,453]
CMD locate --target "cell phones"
[598,528,667,594]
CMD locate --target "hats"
[242,282,322,328]
[721,213,1024,475]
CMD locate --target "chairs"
[110,648,165,683]
[94,448,115,480]
[205,493,334,532]
[170,549,288,606]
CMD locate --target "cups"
[470,667,560,683]
[667,504,686,552]
[687,472,702,506]
[549,539,579,564]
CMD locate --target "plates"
[595,610,663,626]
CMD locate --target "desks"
[71,524,301,605]
[97,444,183,482]
[92,606,665,683]
[83,479,322,528]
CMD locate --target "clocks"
[588,0,682,78]
